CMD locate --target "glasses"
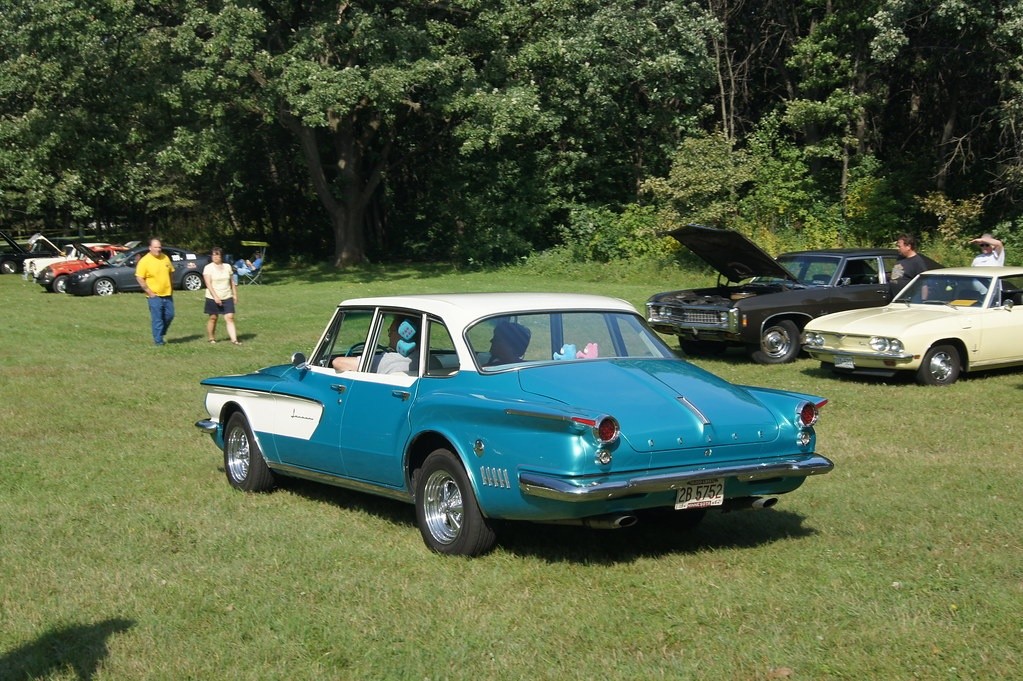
[980,244,990,248]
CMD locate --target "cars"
[63,245,212,298]
[802,266,1022,385]
[0,231,52,274]
[195,294,837,562]
[644,224,1022,365]
[36,241,143,294]
[23,233,123,283]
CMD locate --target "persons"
[134,239,176,348]
[889,233,928,303]
[231,250,262,285]
[484,321,530,367]
[970,232,1006,307]
[200,246,242,347]
[331,314,444,374]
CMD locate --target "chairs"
[377,351,442,377]
[812,274,833,286]
[243,262,263,287]
[476,352,495,369]
[958,290,982,303]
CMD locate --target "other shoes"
[231,341,242,346]
[208,338,216,343]
[232,266,237,272]
[156,342,167,346]
